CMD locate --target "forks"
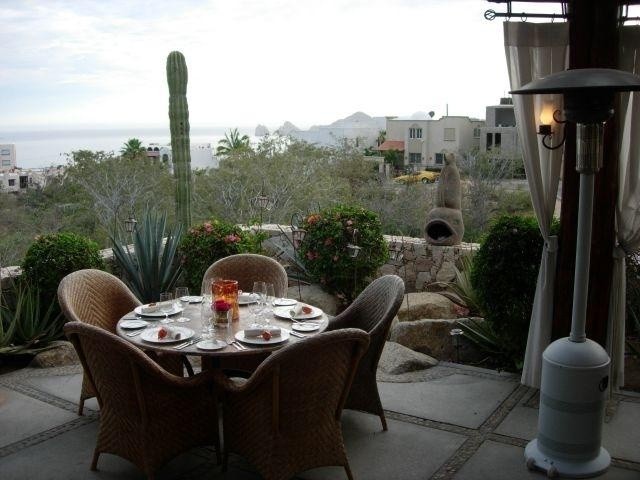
[225,336,248,351]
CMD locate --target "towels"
[162,326,181,339]
[290,304,302,317]
[141,302,172,313]
[238,295,255,301]
[244,328,281,337]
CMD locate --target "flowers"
[298,204,390,305]
[211,300,232,319]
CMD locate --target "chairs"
[322,273,406,431]
[58,268,185,417]
[222,328,371,480]
[64,321,222,480]
[200,253,288,378]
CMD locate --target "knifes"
[123,317,161,321]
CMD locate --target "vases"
[212,311,228,323]
[336,304,351,315]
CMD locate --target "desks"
[116,293,330,379]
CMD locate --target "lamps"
[535,101,566,150]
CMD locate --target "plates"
[274,305,323,319]
[237,295,259,305]
[274,299,297,305]
[180,295,203,304]
[292,322,320,332]
[134,303,183,317]
[196,340,227,351]
[141,326,195,343]
[119,320,147,328]
[235,328,290,345]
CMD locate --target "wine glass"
[175,287,190,323]
[249,282,275,328]
[159,293,174,323]
[201,296,216,338]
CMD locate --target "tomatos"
[158,330,166,339]
[302,307,311,314]
[263,332,271,340]
